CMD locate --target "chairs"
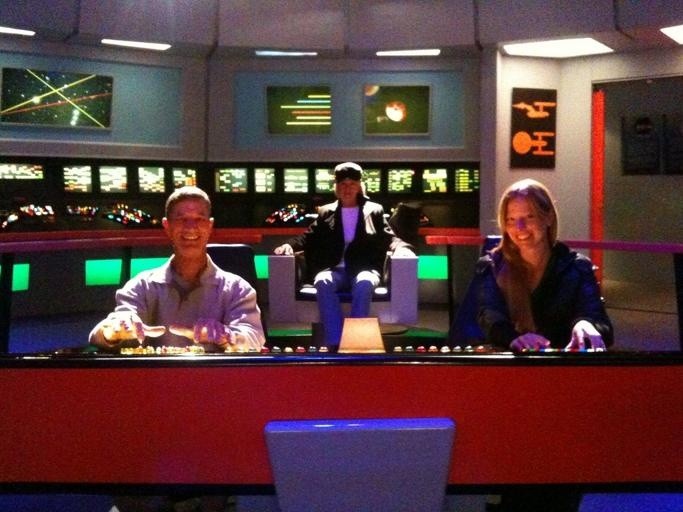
[265,245,420,347]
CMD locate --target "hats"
[333,161,363,181]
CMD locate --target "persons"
[448,180,615,353]
[85,184,268,352]
[269,161,398,347]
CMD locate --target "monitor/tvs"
[263,80,334,137]
[0,67,114,132]
[361,80,433,138]
